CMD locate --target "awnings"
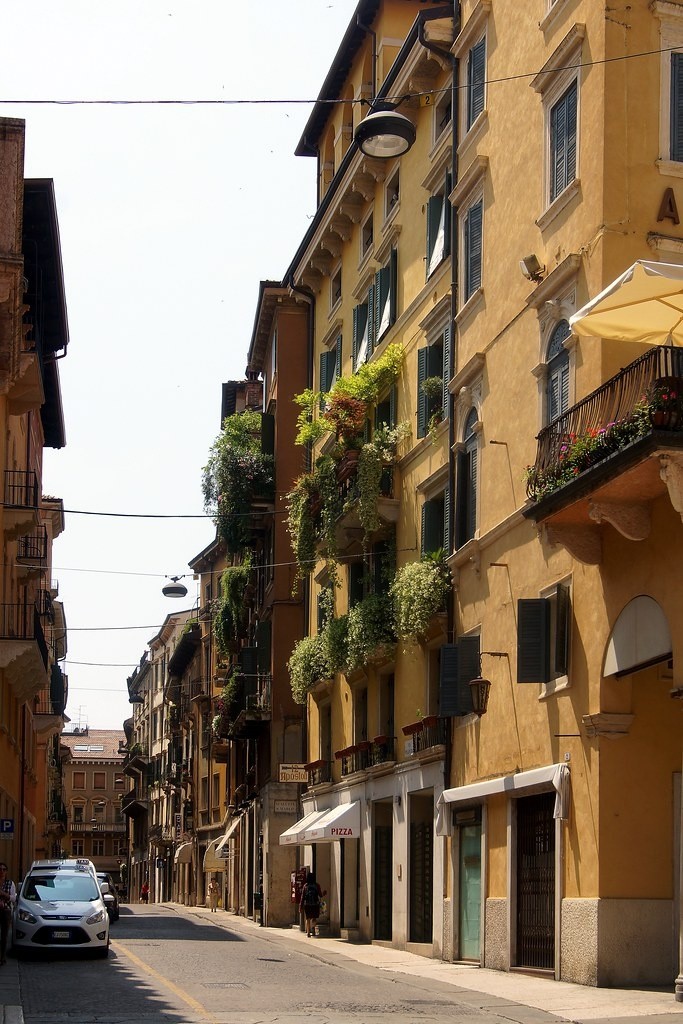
[278,799,360,846]
[174,842,192,863]
[203,818,239,872]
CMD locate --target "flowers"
[524,385,679,503]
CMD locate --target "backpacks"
[305,884,318,904]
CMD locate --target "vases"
[402,714,438,735]
[375,735,388,744]
[334,741,370,759]
[303,759,327,774]
[653,408,678,426]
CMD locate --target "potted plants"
[327,395,367,461]
[421,375,446,446]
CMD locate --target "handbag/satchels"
[0,899,5,908]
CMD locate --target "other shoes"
[308,934,311,938]
[312,928,315,936]
[1,953,7,966]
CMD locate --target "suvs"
[18,857,109,896]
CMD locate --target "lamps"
[163,577,187,598]
[467,652,507,719]
[239,802,251,809]
[352,99,418,157]
[520,254,546,285]
[230,809,243,816]
[128,691,145,705]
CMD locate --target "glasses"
[0,868,7,871]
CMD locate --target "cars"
[6,867,116,960]
[96,872,119,925]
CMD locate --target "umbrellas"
[568,259,683,347]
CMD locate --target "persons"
[208,877,219,912]
[300,873,326,938]
[140,881,149,904]
